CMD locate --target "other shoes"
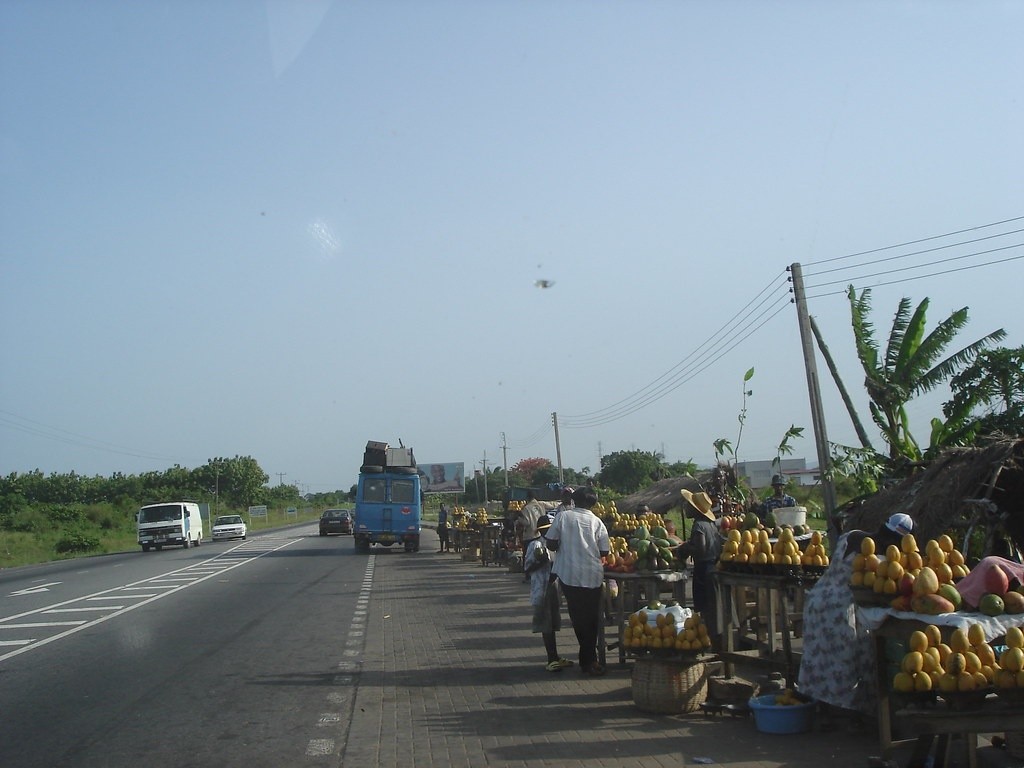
[580,662,605,677]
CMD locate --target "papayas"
[888,564,1024,616]
[627,525,680,570]
[646,600,680,610]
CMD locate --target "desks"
[444,513,1024,768]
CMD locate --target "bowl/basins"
[748,693,818,735]
[772,506,807,528]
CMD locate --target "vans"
[135,501,203,551]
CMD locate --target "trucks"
[353,472,424,551]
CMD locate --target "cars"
[211,515,247,542]
[319,509,354,536]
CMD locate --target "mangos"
[620,614,712,650]
[892,621,1024,692]
[717,514,829,567]
[849,534,970,595]
[592,500,666,566]
[446,506,488,530]
[508,500,526,511]
[773,687,808,706]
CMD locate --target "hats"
[537,514,555,529]
[771,475,788,486]
[885,513,913,536]
[681,489,716,522]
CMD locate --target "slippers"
[546,661,560,671]
[559,657,573,667]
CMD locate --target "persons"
[518,490,543,583]
[524,514,574,672]
[678,489,723,650]
[557,488,574,516]
[797,513,914,714]
[758,475,797,522]
[544,487,610,676]
[437,503,449,553]
[420,489,425,513]
